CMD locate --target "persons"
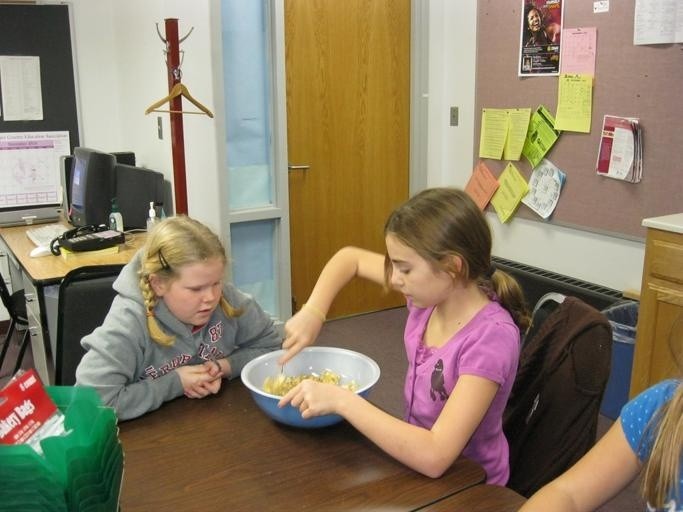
[512,374,683,512]
[523,4,559,56]
[63,209,284,429]
[275,180,523,494]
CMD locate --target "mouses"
[30,247,54,257]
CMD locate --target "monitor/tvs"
[69,147,163,228]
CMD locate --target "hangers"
[142,69,211,116]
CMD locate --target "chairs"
[1,259,31,377]
[55,263,128,387]
[502,291,614,498]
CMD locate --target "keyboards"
[26,224,70,247]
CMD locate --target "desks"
[414,483,528,512]
[117,376,486,512]
[1,222,146,384]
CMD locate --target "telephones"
[56,224,125,253]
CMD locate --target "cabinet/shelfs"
[628,210,682,400]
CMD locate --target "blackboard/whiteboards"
[0,1,84,211]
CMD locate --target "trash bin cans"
[598,300,638,421]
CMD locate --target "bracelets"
[297,300,330,324]
[208,359,225,377]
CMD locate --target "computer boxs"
[59,152,135,224]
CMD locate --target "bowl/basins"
[241,345,381,429]
